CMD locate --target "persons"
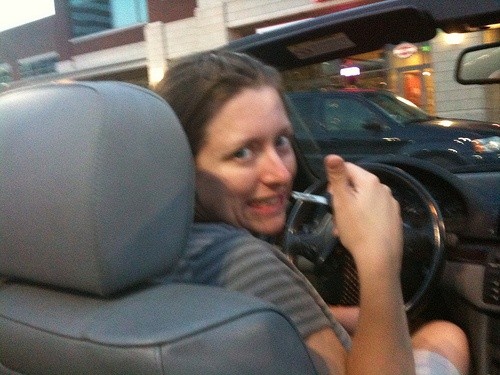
[151,48,471,375]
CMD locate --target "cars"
[283,87,500,174]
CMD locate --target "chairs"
[2,79,318,374]
